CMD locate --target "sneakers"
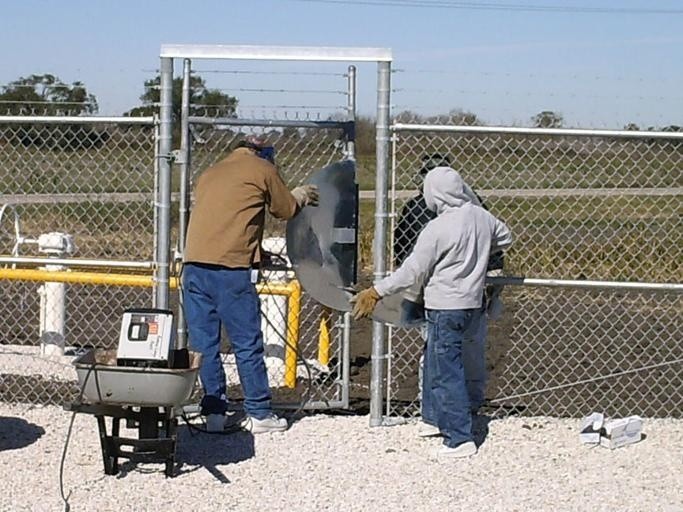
[433,440,478,458]
[206,413,228,432]
[416,420,443,437]
[247,412,289,434]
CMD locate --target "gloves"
[348,286,383,322]
[289,184,321,208]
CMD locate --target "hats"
[420,152,451,176]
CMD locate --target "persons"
[394,154,502,415]
[349,166,512,457]
[181,141,319,433]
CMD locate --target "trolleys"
[68,344,207,482]
